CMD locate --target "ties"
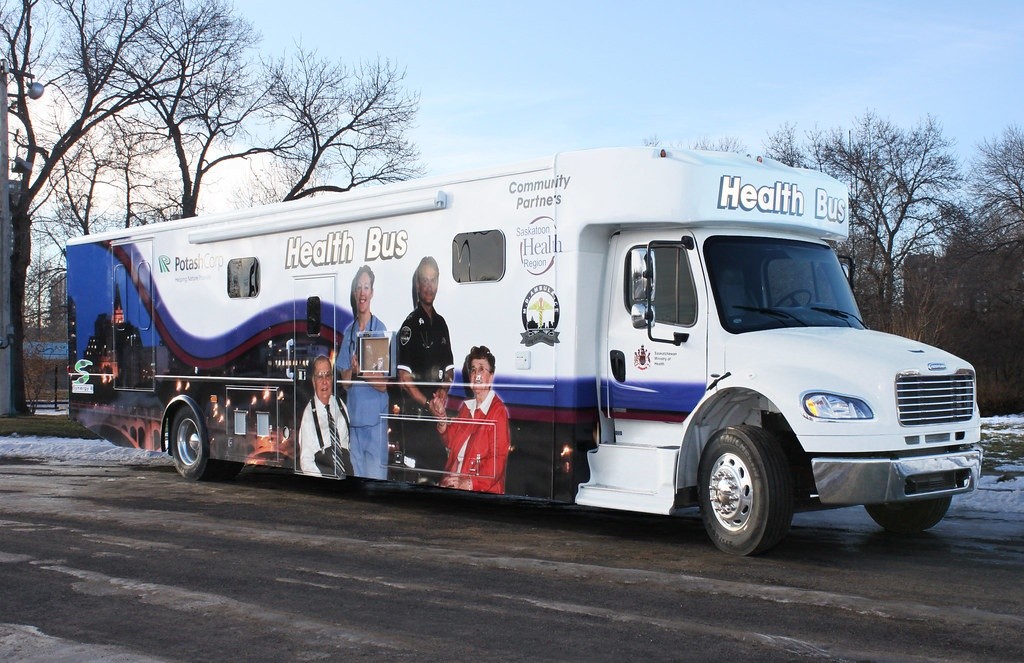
[324,403,346,478]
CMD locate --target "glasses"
[314,372,334,379]
[470,366,491,376]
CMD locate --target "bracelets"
[425,399,430,412]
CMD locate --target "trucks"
[65,148,983,555]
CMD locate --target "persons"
[336,266,389,481]
[397,257,454,484]
[299,354,354,477]
[433,346,509,494]
